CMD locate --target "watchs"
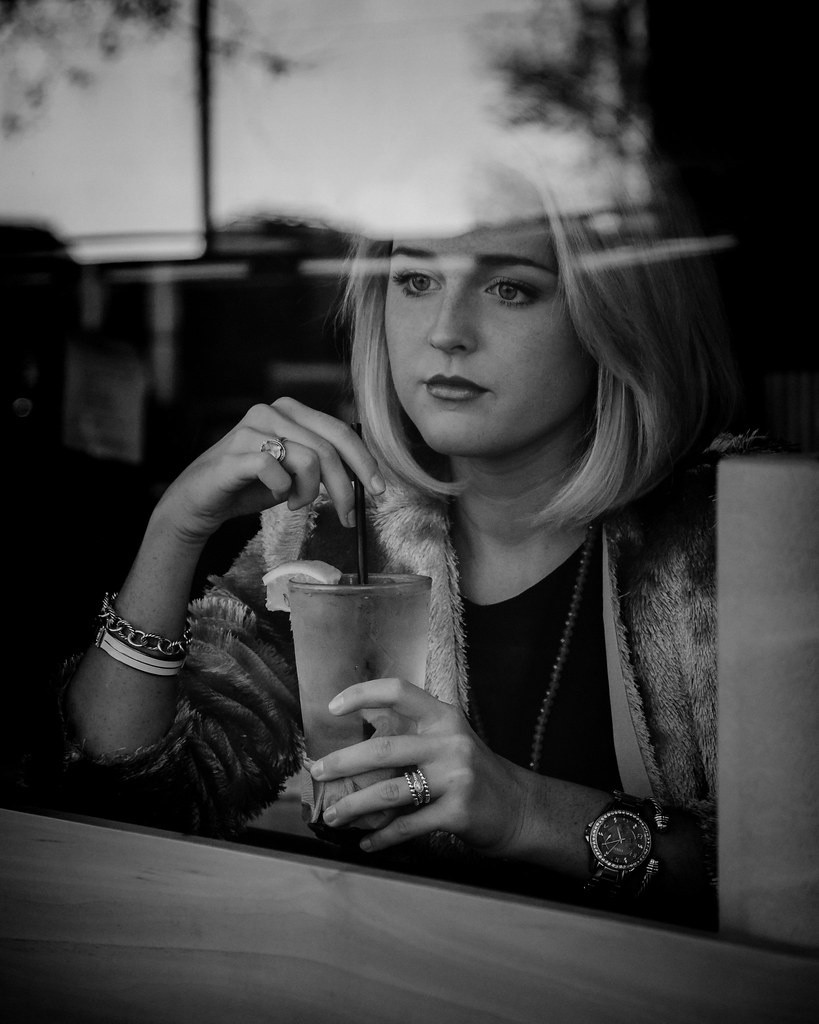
[584,791,669,903]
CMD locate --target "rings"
[260,436,287,462]
[405,770,430,806]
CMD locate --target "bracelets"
[93,592,188,677]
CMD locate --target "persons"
[0,223,235,805]
[56,98,720,932]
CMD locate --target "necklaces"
[469,525,595,771]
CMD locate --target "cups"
[288,572,432,828]
